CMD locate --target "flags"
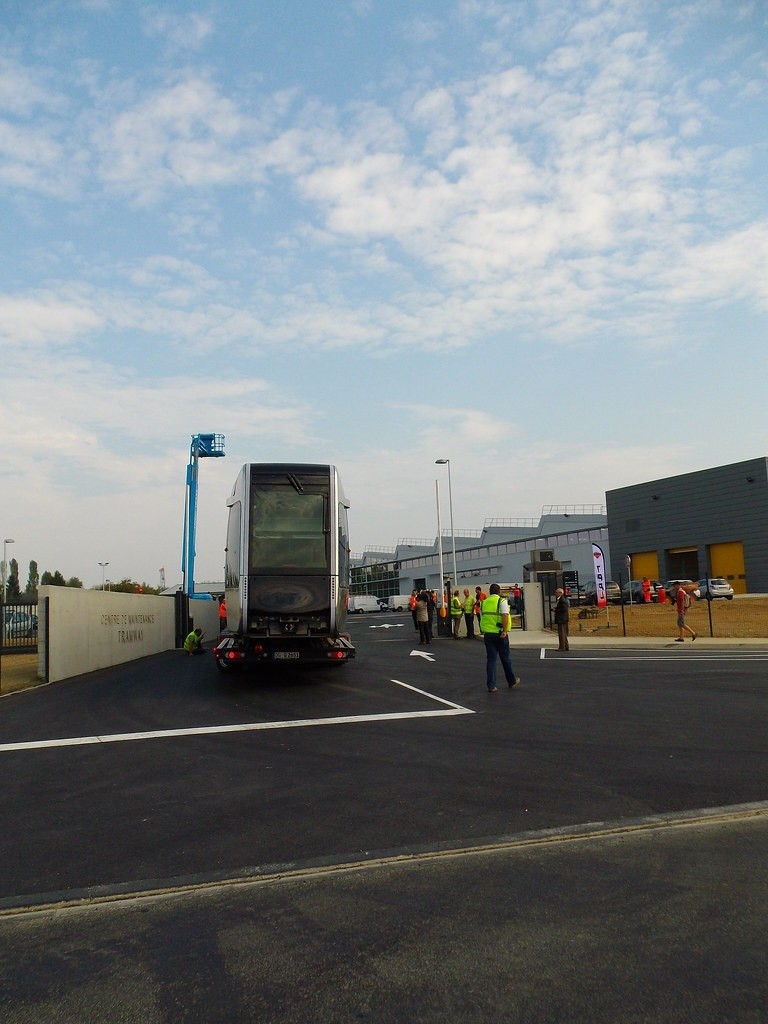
[591,544,607,608]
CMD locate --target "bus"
[214,462,356,673]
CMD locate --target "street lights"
[435,459,458,585]
[4,539,16,603]
[98,562,110,591]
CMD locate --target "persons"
[552,588,569,651]
[475,587,487,635]
[673,581,697,641]
[184,629,207,656]
[642,577,651,603]
[411,589,437,644]
[479,583,520,692]
[509,584,521,614]
[220,599,227,630]
[451,590,464,639]
[463,588,476,639]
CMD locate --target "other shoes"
[674,638,684,642]
[426,641,431,644]
[692,633,698,641]
[555,649,569,651]
[489,687,498,691]
[418,642,425,645]
[512,677,520,687]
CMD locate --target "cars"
[566,589,587,605]
[622,580,665,604]
[697,579,734,601]
[3,612,33,639]
[31,615,38,629]
[582,580,621,606]
[664,580,700,605]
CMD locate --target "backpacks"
[680,589,692,608]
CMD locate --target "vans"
[350,598,388,614]
[389,595,412,612]
[420,589,442,608]
